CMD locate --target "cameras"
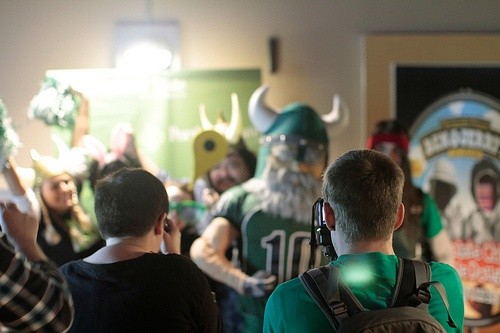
[317,199,338,262]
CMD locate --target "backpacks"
[297,258,448,333]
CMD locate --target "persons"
[263,149,464,333]
[0,193,73,333]
[31,92,257,299]
[368,119,454,270]
[189,84,348,333]
[58,168,223,333]
[461,174,500,245]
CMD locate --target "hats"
[29,149,93,245]
